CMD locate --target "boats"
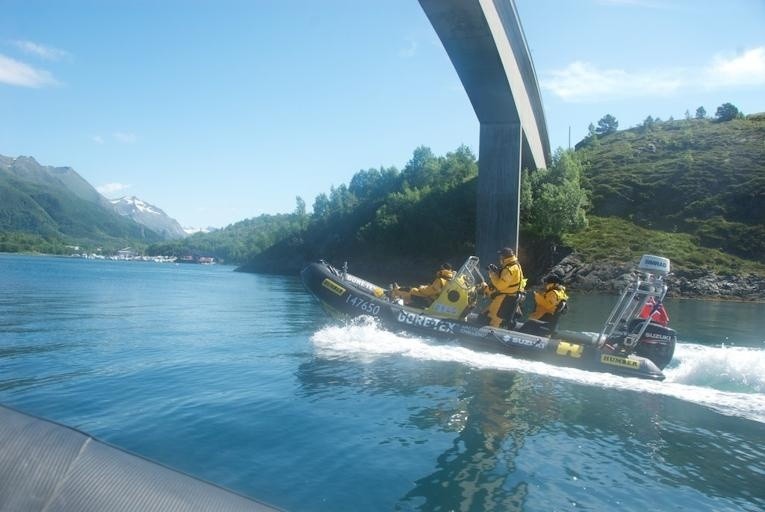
[297,255,677,381]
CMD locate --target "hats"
[496,247,513,256]
[544,275,560,283]
[441,262,451,270]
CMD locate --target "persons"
[488,247,522,327]
[517,273,570,337]
[392,263,453,308]
[478,279,528,318]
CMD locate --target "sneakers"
[392,282,399,297]
